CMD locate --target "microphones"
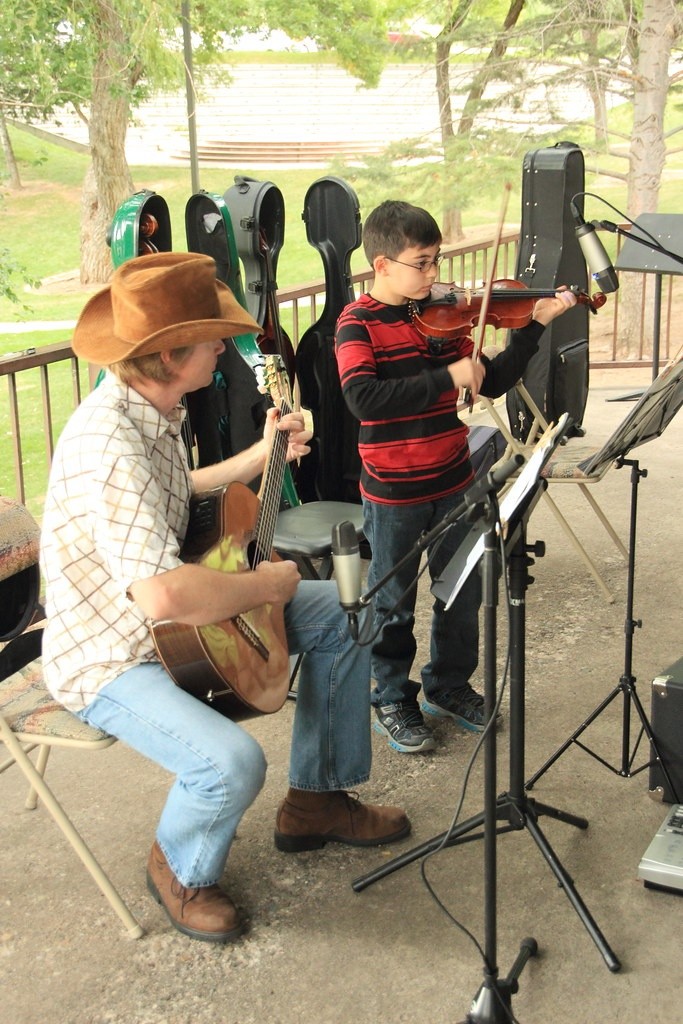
[574,223,619,294]
[331,520,363,613]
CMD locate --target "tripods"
[525,353,683,811]
[357,455,539,1023]
[605,214,683,401]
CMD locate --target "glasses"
[385,254,444,272]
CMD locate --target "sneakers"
[421,682,504,732]
[372,699,436,753]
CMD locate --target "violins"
[408,278,606,343]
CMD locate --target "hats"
[70,252,265,365]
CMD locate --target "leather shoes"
[146,840,243,943]
[273,790,411,853]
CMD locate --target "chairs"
[474,372,632,604]
[271,454,368,703]
[0,497,147,941]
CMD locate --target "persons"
[37,250,415,949]
[331,200,580,755]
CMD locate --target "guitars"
[151,353,292,724]
